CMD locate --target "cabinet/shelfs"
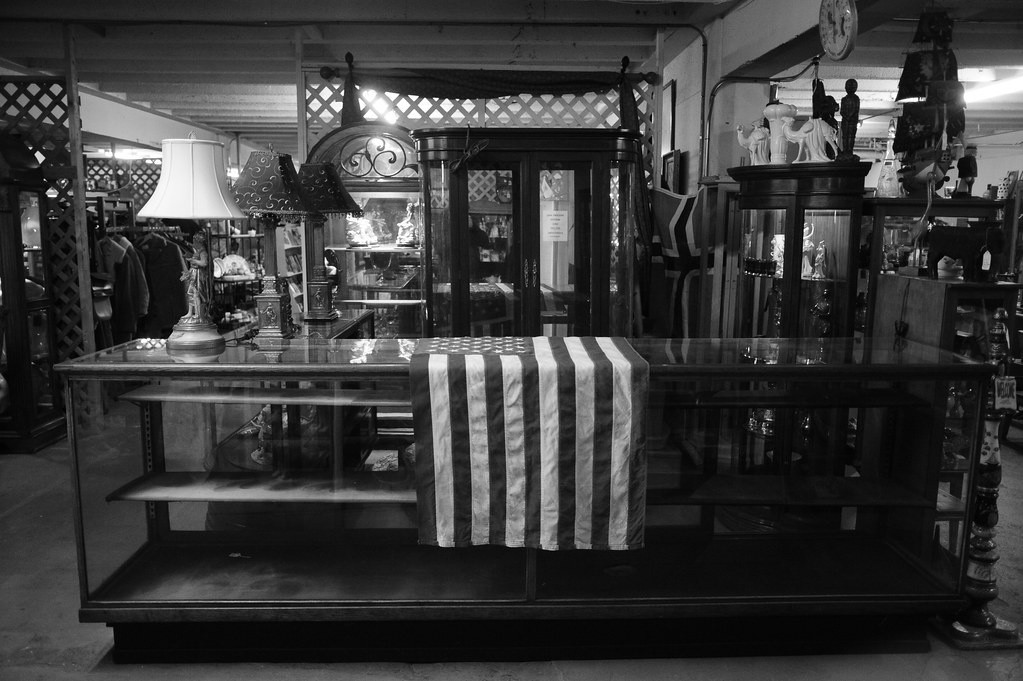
[62,242,979,671]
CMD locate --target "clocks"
[818,0,859,61]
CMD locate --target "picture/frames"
[662,148,681,197]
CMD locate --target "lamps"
[294,164,365,322]
[231,147,312,338]
[137,139,249,352]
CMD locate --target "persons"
[803,251,813,276]
[469,215,491,258]
[212,241,265,308]
[180,235,209,319]
[764,286,780,337]
[840,78,860,156]
[812,248,826,278]
[803,223,814,253]
[957,146,977,194]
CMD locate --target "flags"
[894,12,966,155]
[410,337,651,550]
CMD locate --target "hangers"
[95,222,182,245]
